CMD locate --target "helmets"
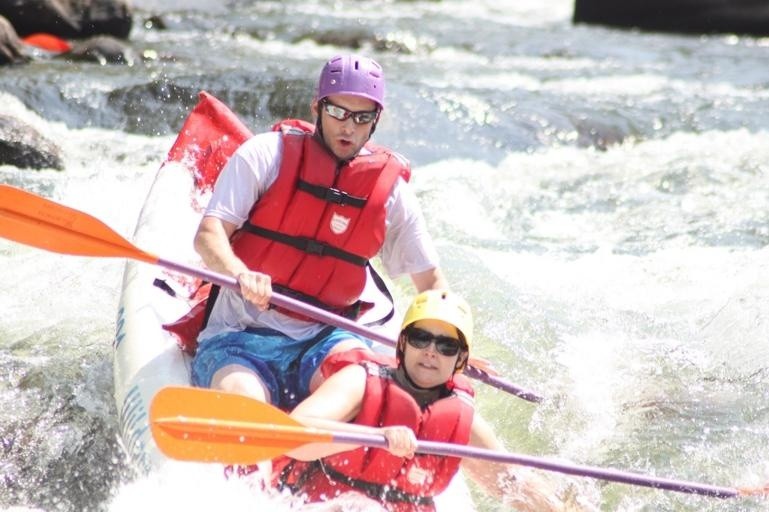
[396,288,475,362]
[316,53,386,110]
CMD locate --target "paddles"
[0,184,547,409]
[150,385,768,499]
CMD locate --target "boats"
[113,91,477,511]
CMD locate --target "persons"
[219,288,561,512]
[190,52,499,419]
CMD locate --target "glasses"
[405,327,461,357]
[321,97,380,125]
